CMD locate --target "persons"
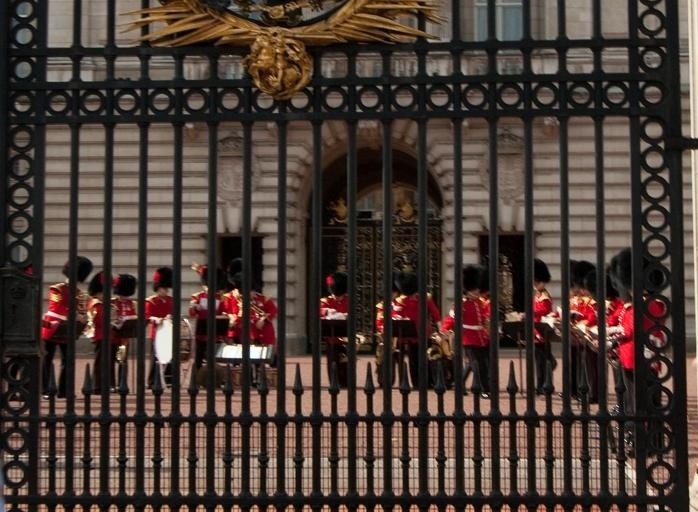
[551,279,669,456]
[372,280,496,394]
[105,293,138,397]
[314,290,354,391]
[528,280,557,395]
[38,270,88,400]
[140,285,181,390]
[185,278,279,400]
[83,287,120,393]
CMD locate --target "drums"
[154,315,193,364]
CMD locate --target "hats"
[201,258,243,292]
[534,257,551,284]
[325,271,346,295]
[63,255,138,297]
[392,271,418,296]
[153,267,173,292]
[569,247,664,302]
[462,265,489,294]
